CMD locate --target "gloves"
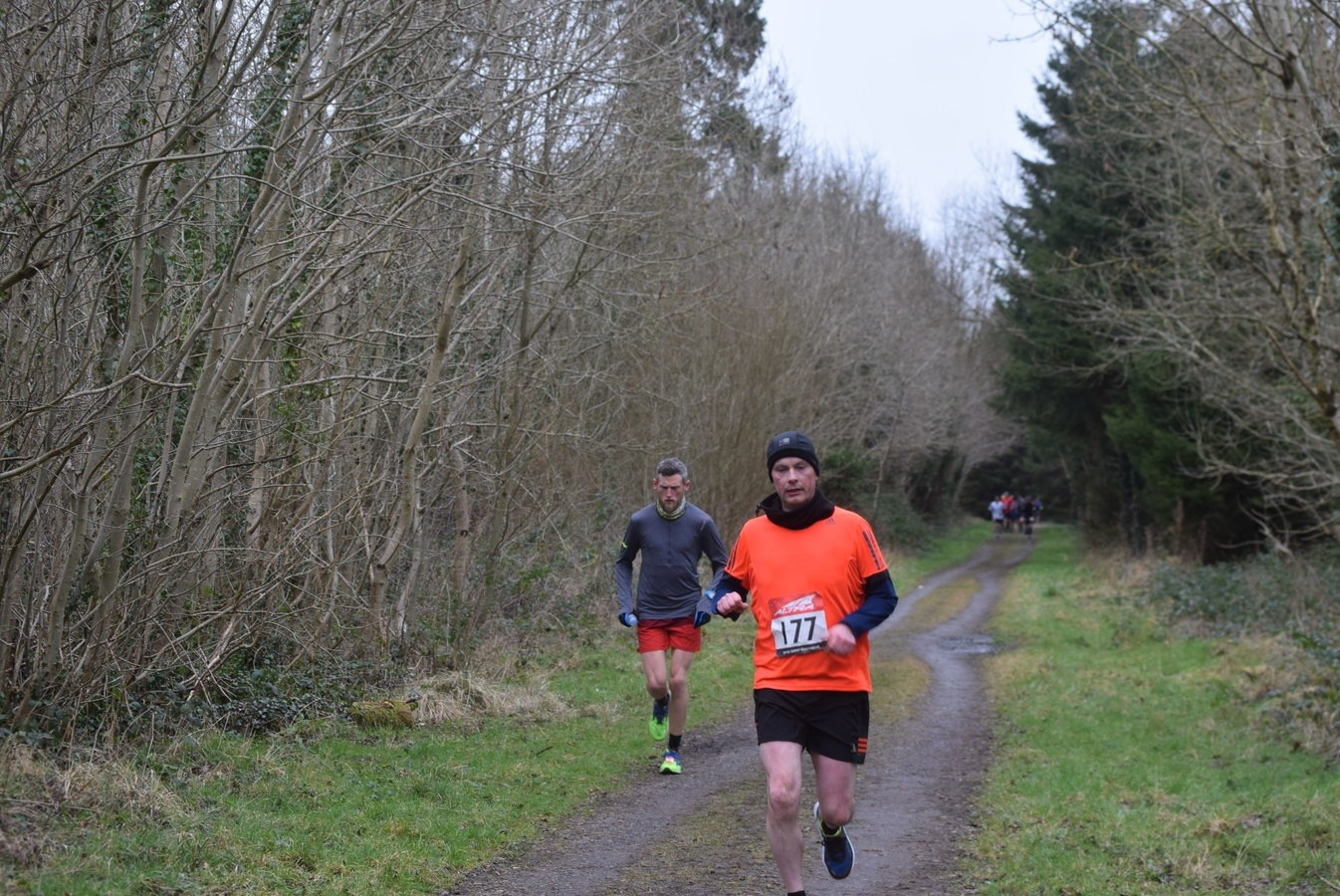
[619,613,631,627]
[694,611,709,628]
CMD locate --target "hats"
[766,429,821,478]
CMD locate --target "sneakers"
[649,689,672,741]
[659,748,680,775]
[814,799,854,880]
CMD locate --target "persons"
[615,456,727,775]
[988,492,1042,536]
[713,431,897,895]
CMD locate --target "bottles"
[627,613,639,627]
